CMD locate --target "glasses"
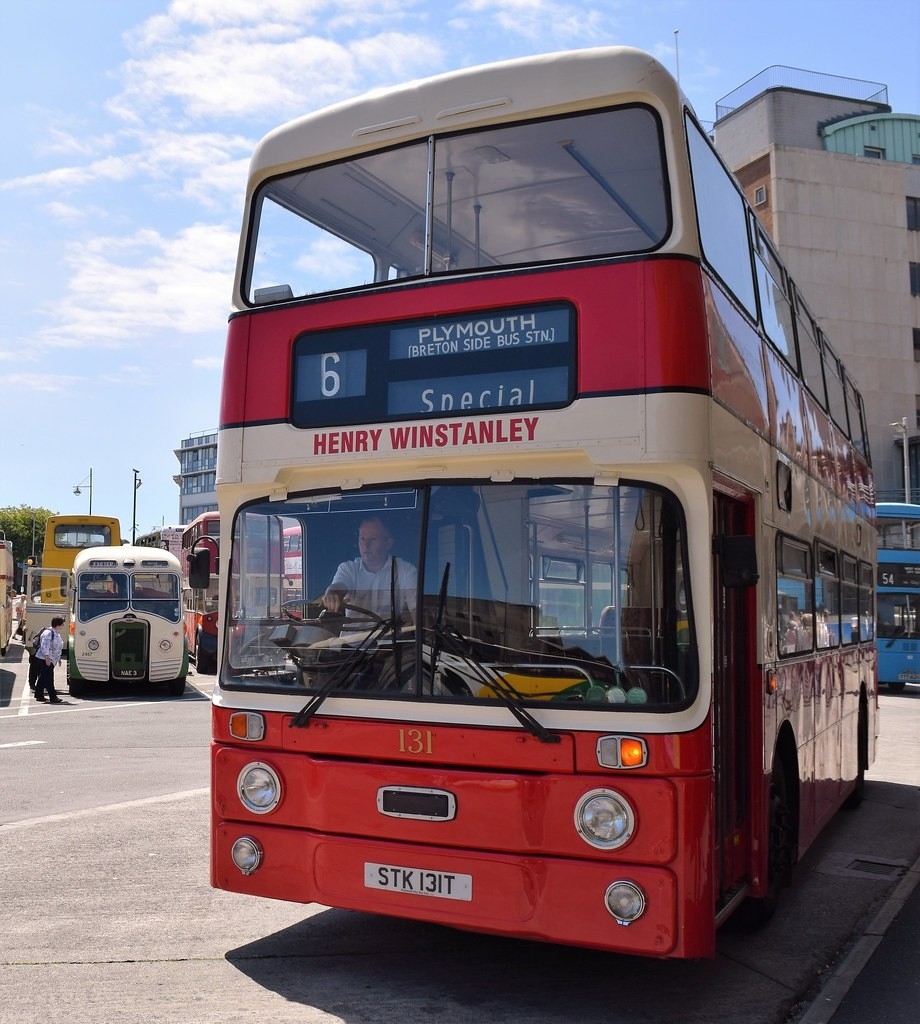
[61,624,64,626]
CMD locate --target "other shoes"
[37,697,49,702]
[12,636,18,640]
[50,696,62,704]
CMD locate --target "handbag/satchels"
[25,627,54,656]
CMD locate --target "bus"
[0,530,15,656]
[25,542,190,696]
[775,501,920,693]
[41,514,121,604]
[182,510,294,677]
[136,524,191,564]
[208,45,879,963]
[282,525,303,619]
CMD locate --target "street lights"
[72,467,92,516]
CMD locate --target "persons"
[13,594,36,641]
[779,609,835,654]
[323,512,430,656]
[29,614,66,704]
[134,583,143,594]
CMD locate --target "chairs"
[598,606,681,678]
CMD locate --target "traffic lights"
[28,556,37,566]
